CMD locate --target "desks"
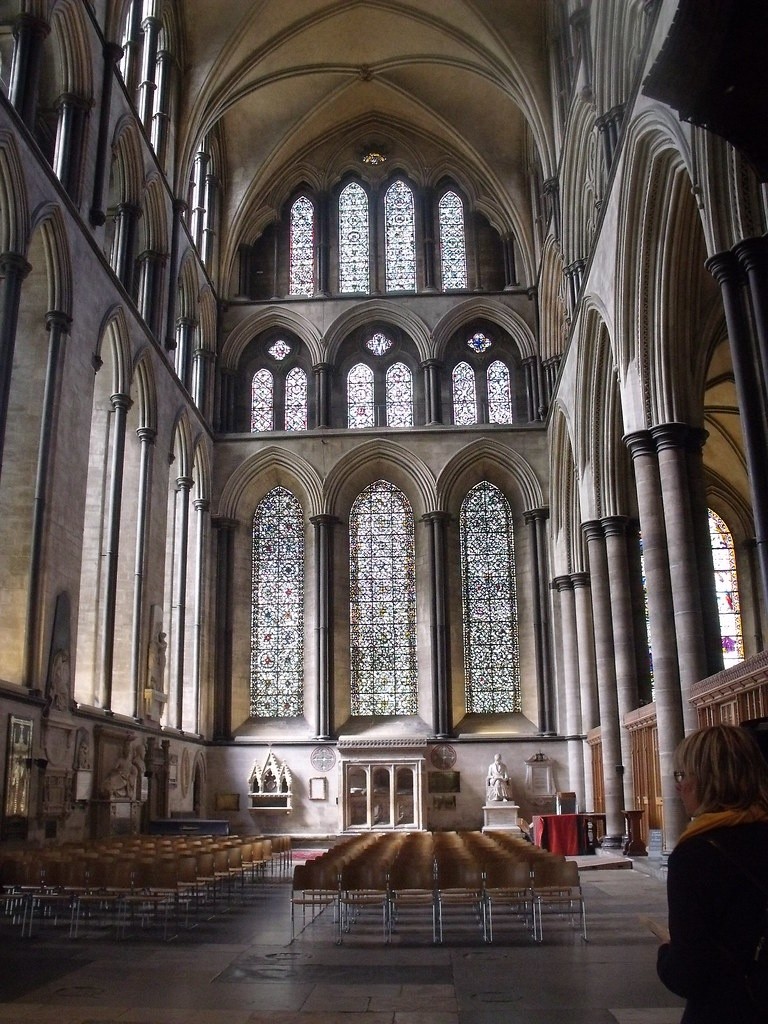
[620,810,648,856]
[533,814,585,856]
[581,813,606,845]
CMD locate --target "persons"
[657,724,768,1024]
[486,753,513,802]
[155,632,167,694]
[52,650,71,712]
[132,744,146,777]
[99,743,138,801]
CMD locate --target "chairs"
[290,830,589,945]
[0,835,293,941]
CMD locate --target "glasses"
[674,770,685,783]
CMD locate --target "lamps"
[362,143,389,165]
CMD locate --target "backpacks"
[690,835,768,1024]
